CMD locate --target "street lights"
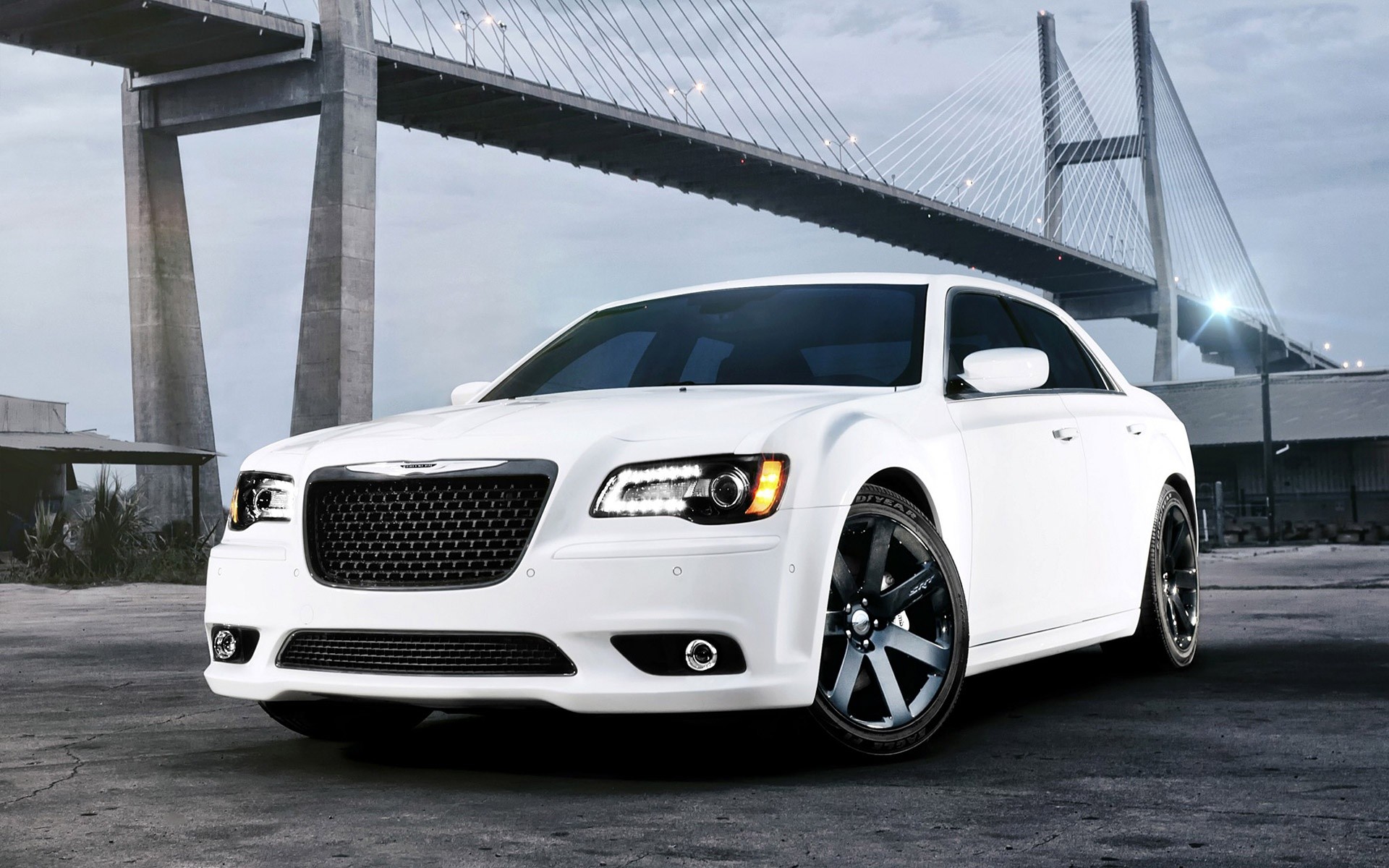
[1211,297,1276,546]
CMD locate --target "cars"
[204,274,1203,769]
